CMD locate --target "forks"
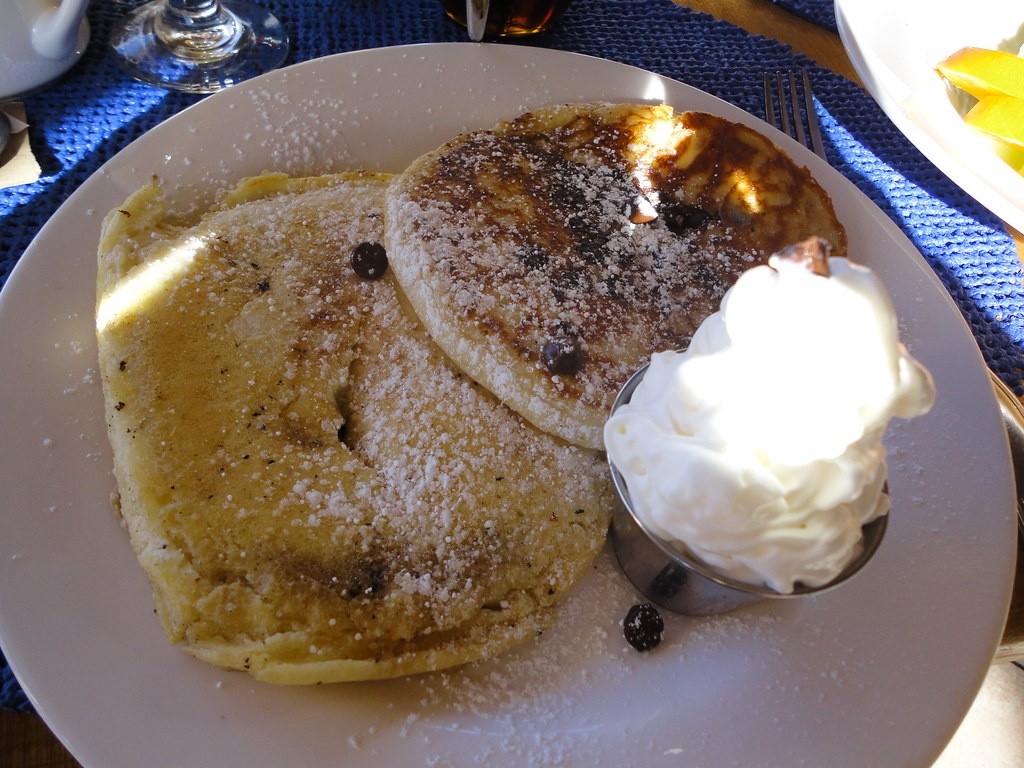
[757,65,1022,451]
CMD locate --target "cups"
[438,2,557,39]
[608,347,888,618]
[0,0,93,98]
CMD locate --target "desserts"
[91,97,919,680]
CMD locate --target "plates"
[0,33,1018,765]
[829,0,1020,232]
